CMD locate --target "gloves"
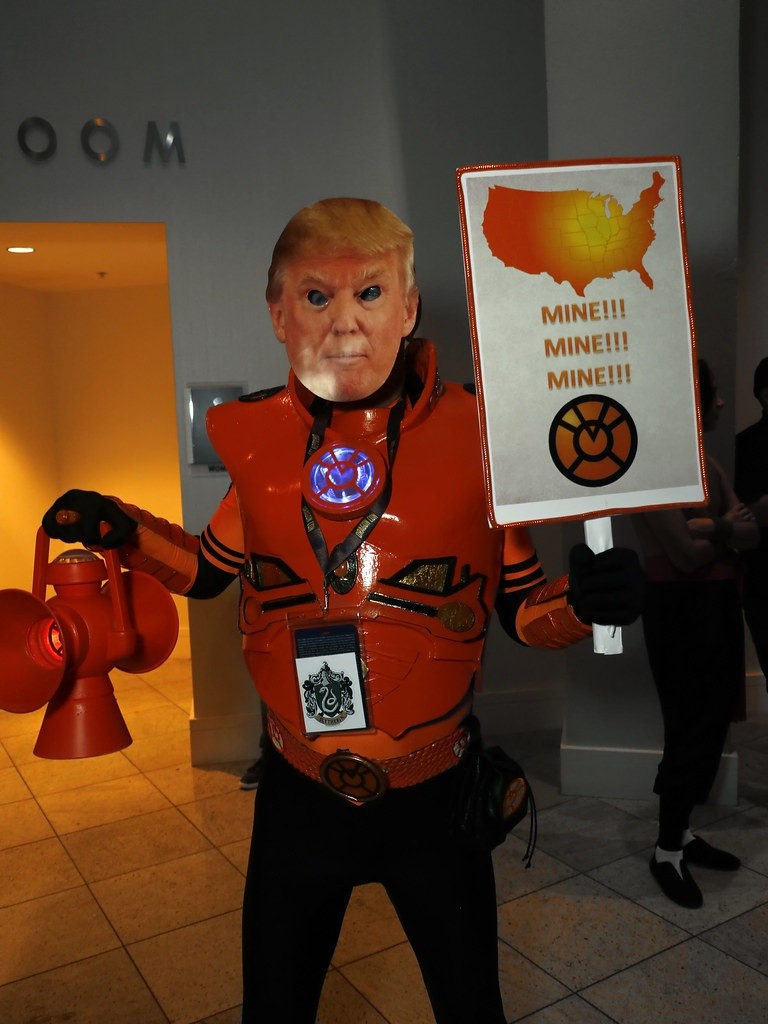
[565,543,643,628]
[42,489,140,550]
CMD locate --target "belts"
[265,705,470,806]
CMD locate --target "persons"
[634,357,757,912]
[732,355,768,691]
[38,196,652,1024]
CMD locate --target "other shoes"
[238,762,264,790]
[680,834,742,871]
[648,853,704,909]
